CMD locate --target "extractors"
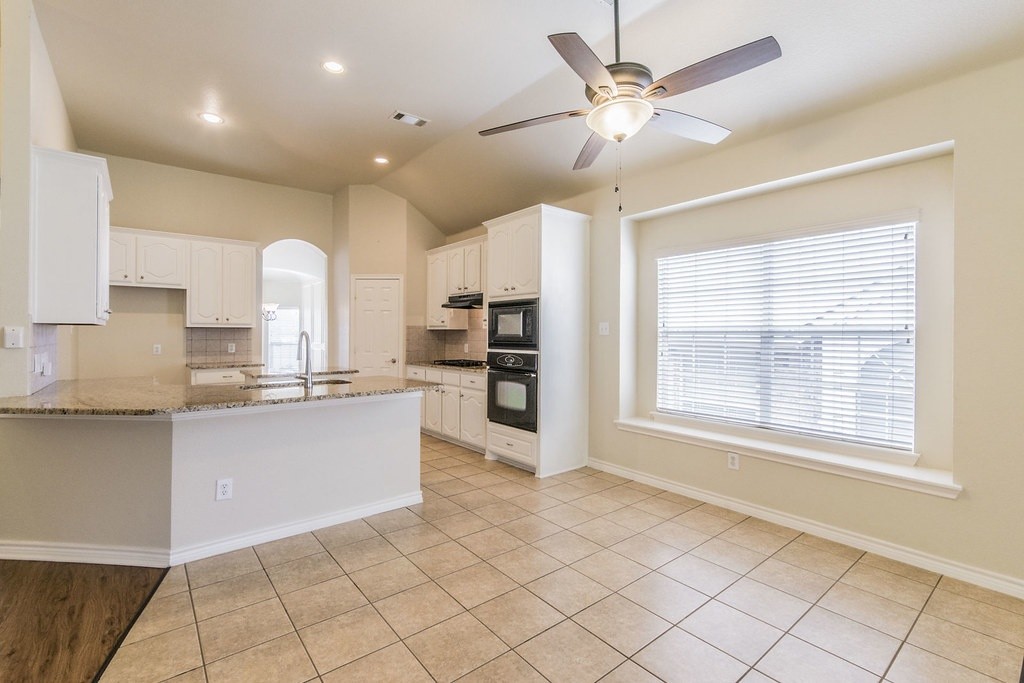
[441,293,483,310]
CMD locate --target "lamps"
[586,97,656,141]
[261,302,280,321]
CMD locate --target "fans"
[479,0,784,170]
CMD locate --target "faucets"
[293,330,313,388]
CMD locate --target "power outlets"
[464,343,468,353]
[154,345,161,354]
[726,451,741,471]
[216,478,231,499]
[229,343,236,352]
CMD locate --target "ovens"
[486,298,539,434]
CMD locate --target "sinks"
[300,379,353,386]
[238,382,307,390]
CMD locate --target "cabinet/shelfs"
[404,367,425,428]
[487,422,538,466]
[428,252,468,330]
[185,235,259,327]
[32,146,115,325]
[425,370,460,439]
[460,374,487,447]
[487,214,541,300]
[109,228,186,290]
[449,243,481,295]
[191,367,260,385]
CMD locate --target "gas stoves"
[432,359,486,368]
